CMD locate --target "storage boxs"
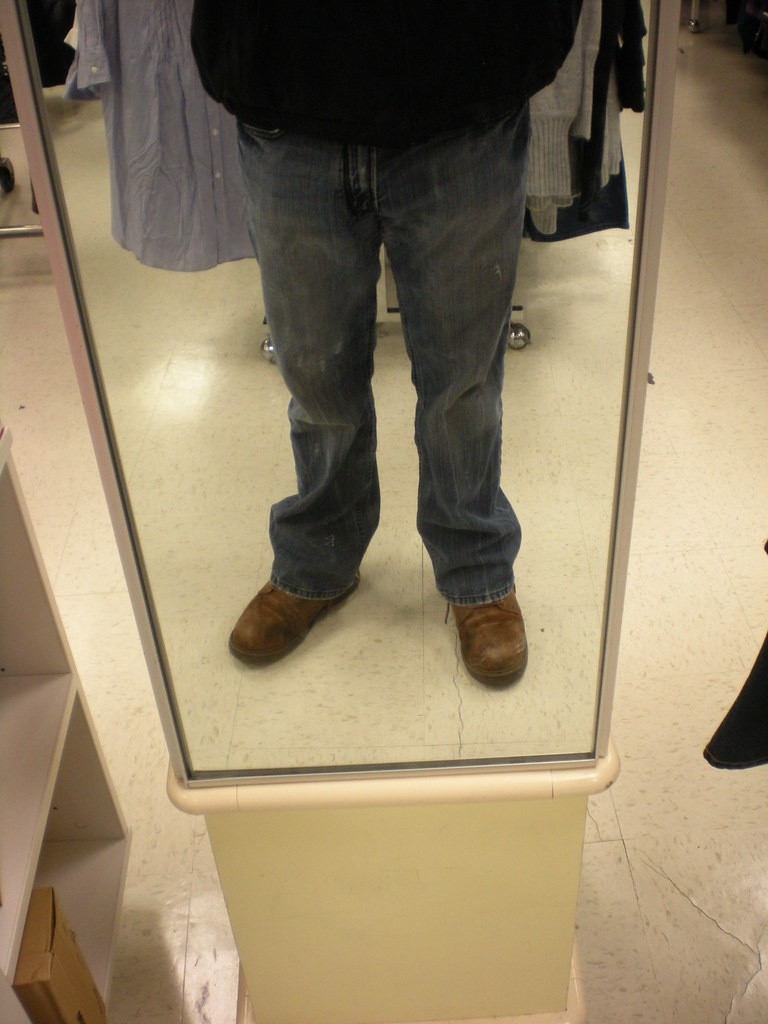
[11,886,107,1024]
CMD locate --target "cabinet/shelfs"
[1,416,136,1024]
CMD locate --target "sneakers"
[443,583,529,679]
[230,569,361,657]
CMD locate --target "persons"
[190,0,583,684]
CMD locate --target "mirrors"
[2,1,666,790]
